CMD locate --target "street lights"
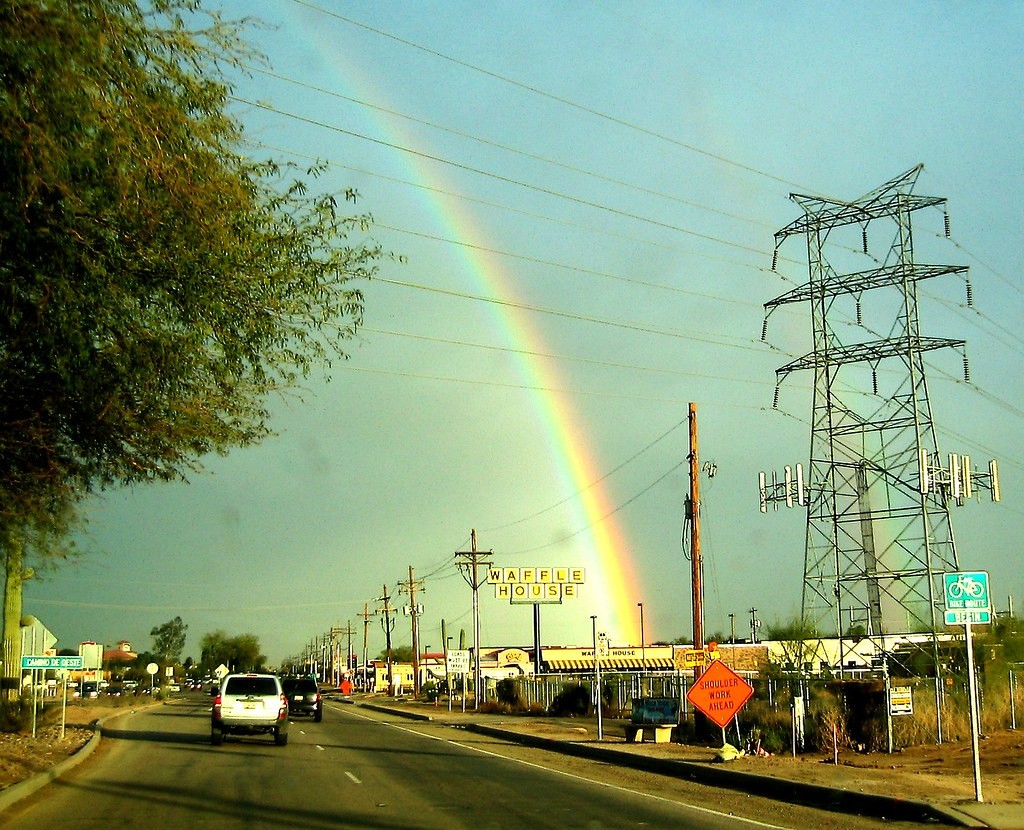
[728,613,735,644]
[637,602,644,647]
[424,645,431,654]
[590,616,597,648]
[447,637,454,649]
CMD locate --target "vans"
[84,680,107,698]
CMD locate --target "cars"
[133,684,161,696]
[184,679,211,691]
[171,683,181,692]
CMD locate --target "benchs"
[619,697,680,743]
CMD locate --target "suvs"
[281,675,323,723]
[211,673,289,745]
